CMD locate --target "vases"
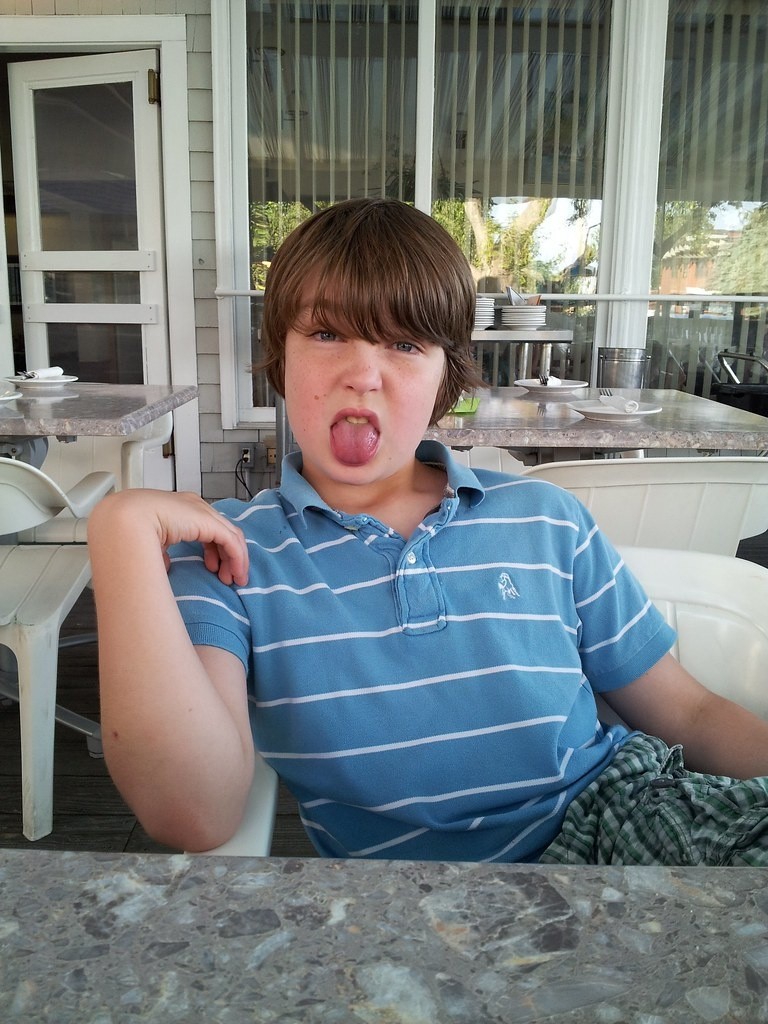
[251,260,272,291]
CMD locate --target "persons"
[87,197,768,868]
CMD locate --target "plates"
[501,305,547,330]
[14,388,80,400]
[4,374,78,388]
[565,399,663,423]
[514,391,583,404]
[474,295,495,330]
[513,378,590,394]
[0,390,24,408]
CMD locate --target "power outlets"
[267,448,277,463]
[238,445,255,468]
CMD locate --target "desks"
[0,849,768,1024]
[471,330,575,378]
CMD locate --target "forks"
[539,374,549,385]
[17,370,34,379]
[599,388,612,397]
[536,401,547,417]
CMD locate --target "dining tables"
[0,382,201,470]
[426,387,768,453]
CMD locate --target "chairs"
[180,543,768,854]
[519,455,768,558]
[1,456,121,845]
[38,412,174,493]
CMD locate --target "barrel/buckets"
[597,345,646,389]
